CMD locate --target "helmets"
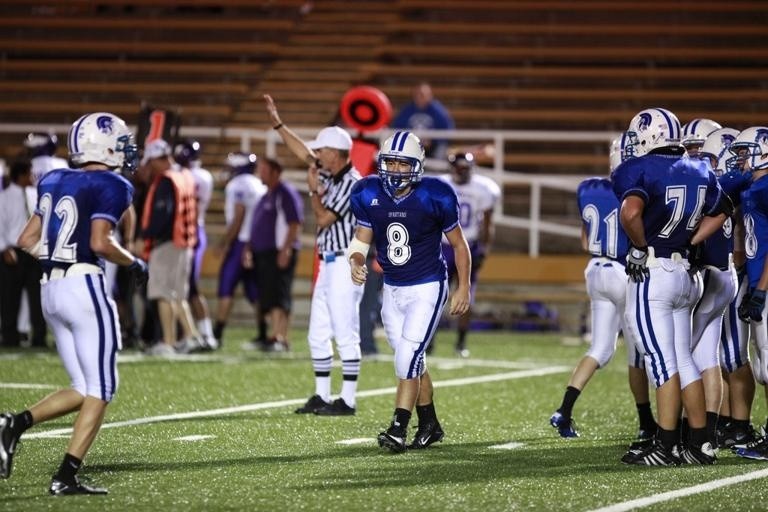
[25,132,58,157]
[68,112,138,172]
[610,107,768,172]
[174,140,198,165]
[378,131,425,192]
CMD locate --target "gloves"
[625,243,650,283]
[738,287,766,322]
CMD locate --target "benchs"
[0,0,768,337]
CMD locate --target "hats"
[305,126,352,150]
[140,140,171,167]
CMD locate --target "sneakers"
[146,335,216,355]
[550,412,580,437]
[295,395,355,415]
[243,335,288,352]
[0,412,20,478]
[50,475,107,495]
[622,422,768,466]
[407,422,444,449]
[377,422,405,453]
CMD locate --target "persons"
[263,82,499,451]
[0,105,218,356]
[0,111,151,494]
[210,151,298,352]
[550,108,768,467]
